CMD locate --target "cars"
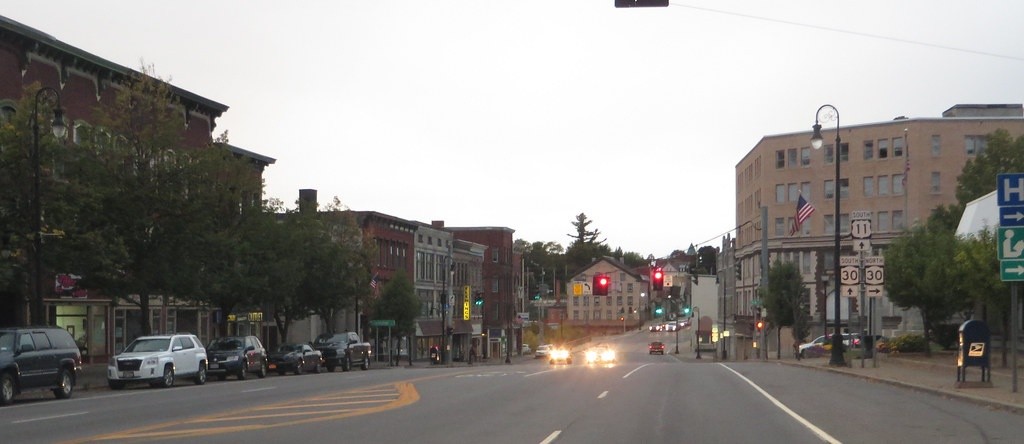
[267,343,324,376]
[549,346,573,364]
[648,342,665,355]
[522,343,532,355]
[648,307,692,332]
[534,344,551,359]
[797,332,866,360]
[586,343,616,364]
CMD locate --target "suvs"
[205,334,269,381]
[0,324,84,407]
[106,330,209,390]
[311,329,372,373]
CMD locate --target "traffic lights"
[653,271,663,291]
[592,275,609,296]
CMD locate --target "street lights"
[440,254,457,365]
[808,103,847,368]
[31,86,72,326]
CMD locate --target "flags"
[901,142,911,185]
[791,195,816,236]
[370,274,379,297]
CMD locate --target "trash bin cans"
[430,347,440,364]
[861,335,881,359]
[957,319,990,382]
